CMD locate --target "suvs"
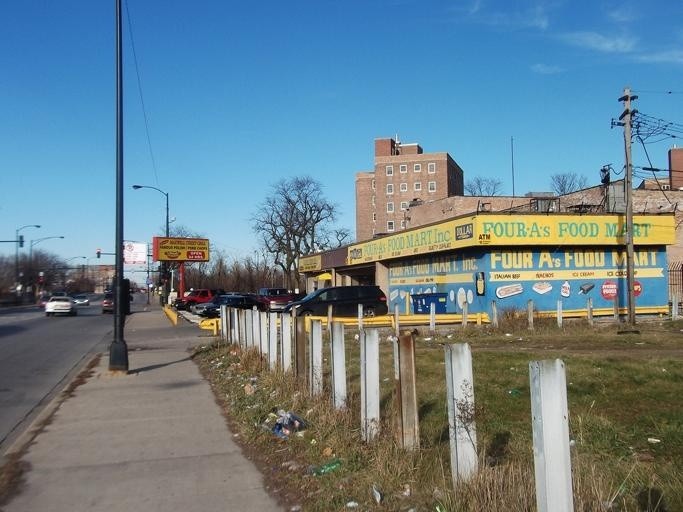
[256,286,294,314]
[101,293,114,314]
[171,288,270,318]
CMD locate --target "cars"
[103,285,146,296]
[2,283,90,308]
[44,295,78,317]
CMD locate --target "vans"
[279,285,388,319]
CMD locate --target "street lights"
[131,184,168,305]
[14,224,41,281]
[28,235,65,271]
[65,255,89,263]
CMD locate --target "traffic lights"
[96,248,100,258]
